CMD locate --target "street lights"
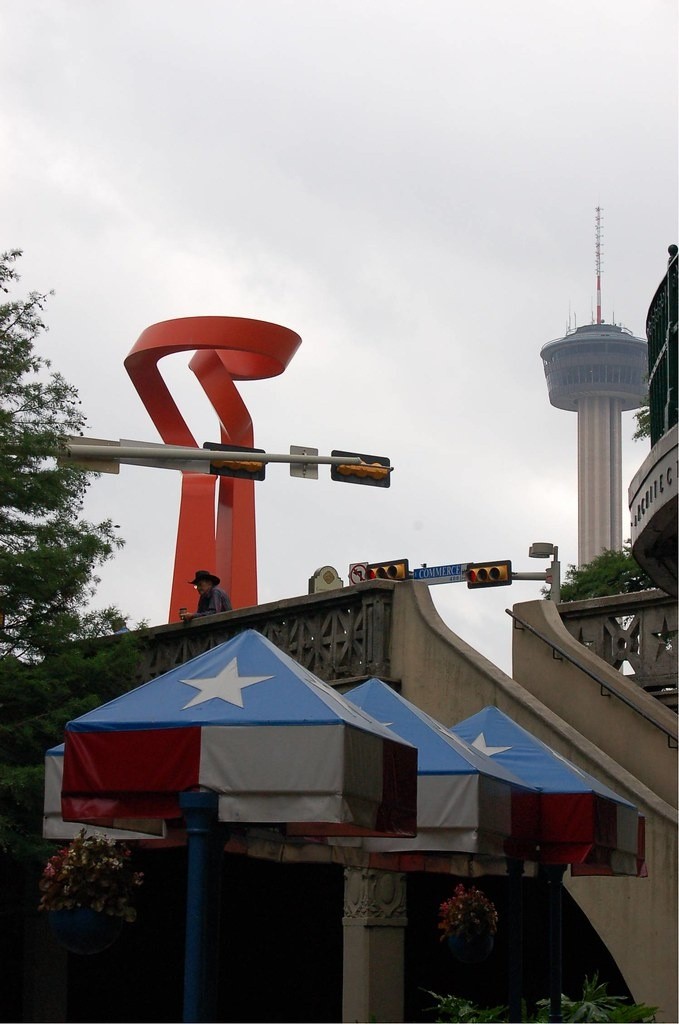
[528,543,561,602]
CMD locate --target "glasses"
[194,583,202,590]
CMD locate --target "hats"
[188,570,220,586]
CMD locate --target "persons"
[111,619,131,635]
[178,570,232,620]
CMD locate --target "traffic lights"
[466,560,511,590]
[365,558,409,580]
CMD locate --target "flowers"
[36,829,144,922]
[438,883,497,944]
